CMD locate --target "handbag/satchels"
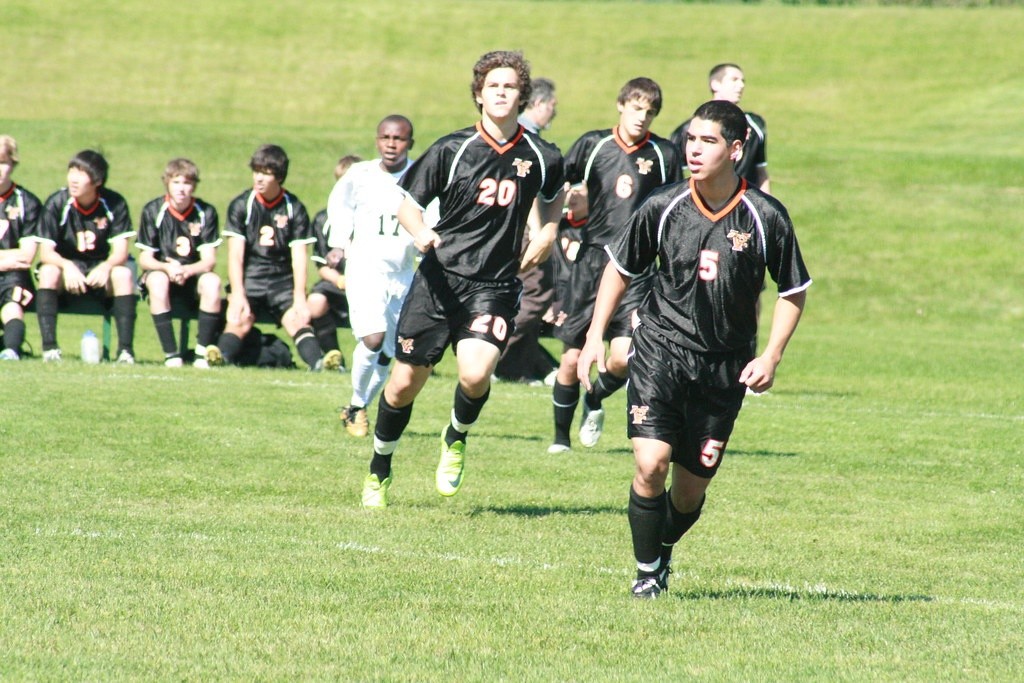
[233,326,297,370]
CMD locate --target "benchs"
[25,254,140,362]
[169,299,553,362]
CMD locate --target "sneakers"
[632,576,661,600]
[435,423,467,497]
[340,403,369,438]
[362,467,393,508]
[579,393,604,448]
[547,444,570,455]
[653,560,673,595]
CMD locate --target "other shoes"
[338,361,347,372]
[116,349,135,365]
[0,347,20,360]
[43,348,62,363]
[163,353,184,368]
[490,373,544,387]
[310,348,342,374]
[192,344,211,370]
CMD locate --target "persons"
[0,134,42,360]
[307,63,768,453]
[32,150,137,364]
[577,99,814,597]
[362,51,571,509]
[134,158,224,370]
[205,145,342,371]
